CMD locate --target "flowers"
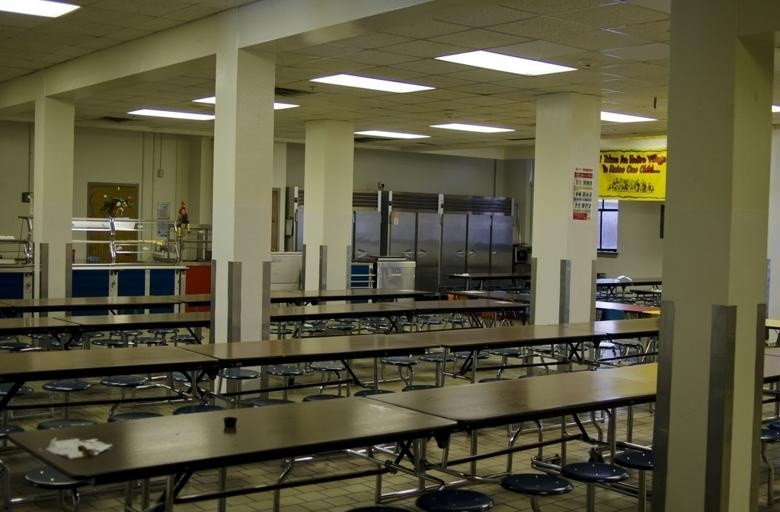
[100,186,135,217]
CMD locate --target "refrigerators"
[270,252,304,290]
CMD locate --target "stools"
[302,328,321,336]
[26,465,87,511]
[171,335,198,345]
[612,339,645,366]
[270,328,293,340]
[402,384,437,392]
[303,392,342,401]
[37,417,96,429]
[416,488,494,510]
[247,399,293,407]
[449,319,464,328]
[108,411,161,421]
[42,380,92,418]
[327,323,355,334]
[422,351,455,388]
[382,357,418,391]
[0,384,33,425]
[144,338,163,347]
[0,423,24,447]
[347,505,413,511]
[100,375,146,419]
[768,421,780,443]
[222,369,259,408]
[530,346,554,376]
[613,449,656,512]
[146,328,178,345]
[311,361,348,396]
[108,330,143,349]
[418,318,440,331]
[479,377,511,382]
[493,349,520,377]
[172,404,224,416]
[455,351,488,376]
[266,364,302,401]
[562,461,628,511]
[355,389,392,396]
[499,474,573,512]
[629,290,652,302]
[760,428,777,506]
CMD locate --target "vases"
[109,217,118,266]
